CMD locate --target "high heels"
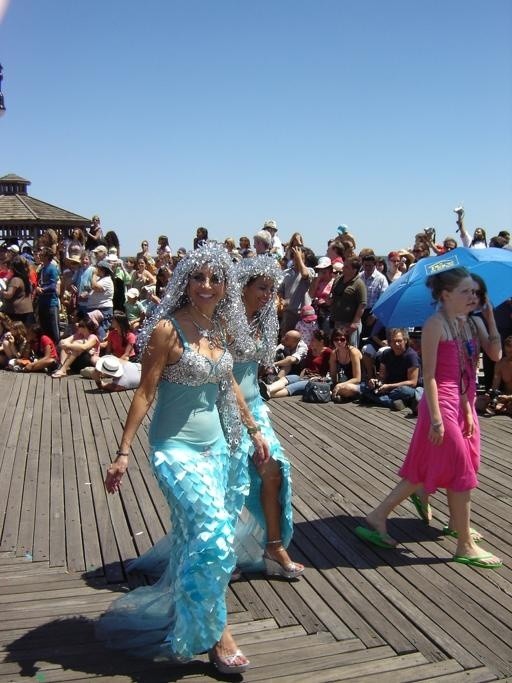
[230,567,242,581]
[263,540,304,578]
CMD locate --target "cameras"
[454,208,464,213]
[401,257,406,263]
[424,228,432,233]
[375,381,381,391]
[488,389,501,408]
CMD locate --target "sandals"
[209,647,249,673]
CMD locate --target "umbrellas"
[369,246,511,328]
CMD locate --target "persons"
[1,216,187,392]
[194,227,208,250]
[254,206,512,419]
[408,271,503,543]
[215,254,305,580]
[96,240,270,675]
[354,263,504,569]
[225,236,254,263]
[114,449,132,456]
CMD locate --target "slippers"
[443,526,483,543]
[355,527,397,548]
[454,552,502,568]
[410,493,433,521]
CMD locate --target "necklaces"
[186,316,225,350]
[439,307,465,376]
[239,317,264,344]
[458,313,482,370]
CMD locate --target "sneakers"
[259,380,270,401]
[50,370,67,378]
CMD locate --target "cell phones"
[480,294,485,306]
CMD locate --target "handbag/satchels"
[303,380,330,403]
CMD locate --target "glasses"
[334,338,345,342]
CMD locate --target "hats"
[91,245,108,252]
[262,220,277,231]
[6,245,19,253]
[314,257,331,268]
[398,249,414,261]
[65,255,81,265]
[125,288,139,299]
[97,261,114,274]
[96,355,124,377]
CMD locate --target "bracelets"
[246,424,263,435]
[430,421,443,430]
[486,334,501,343]
